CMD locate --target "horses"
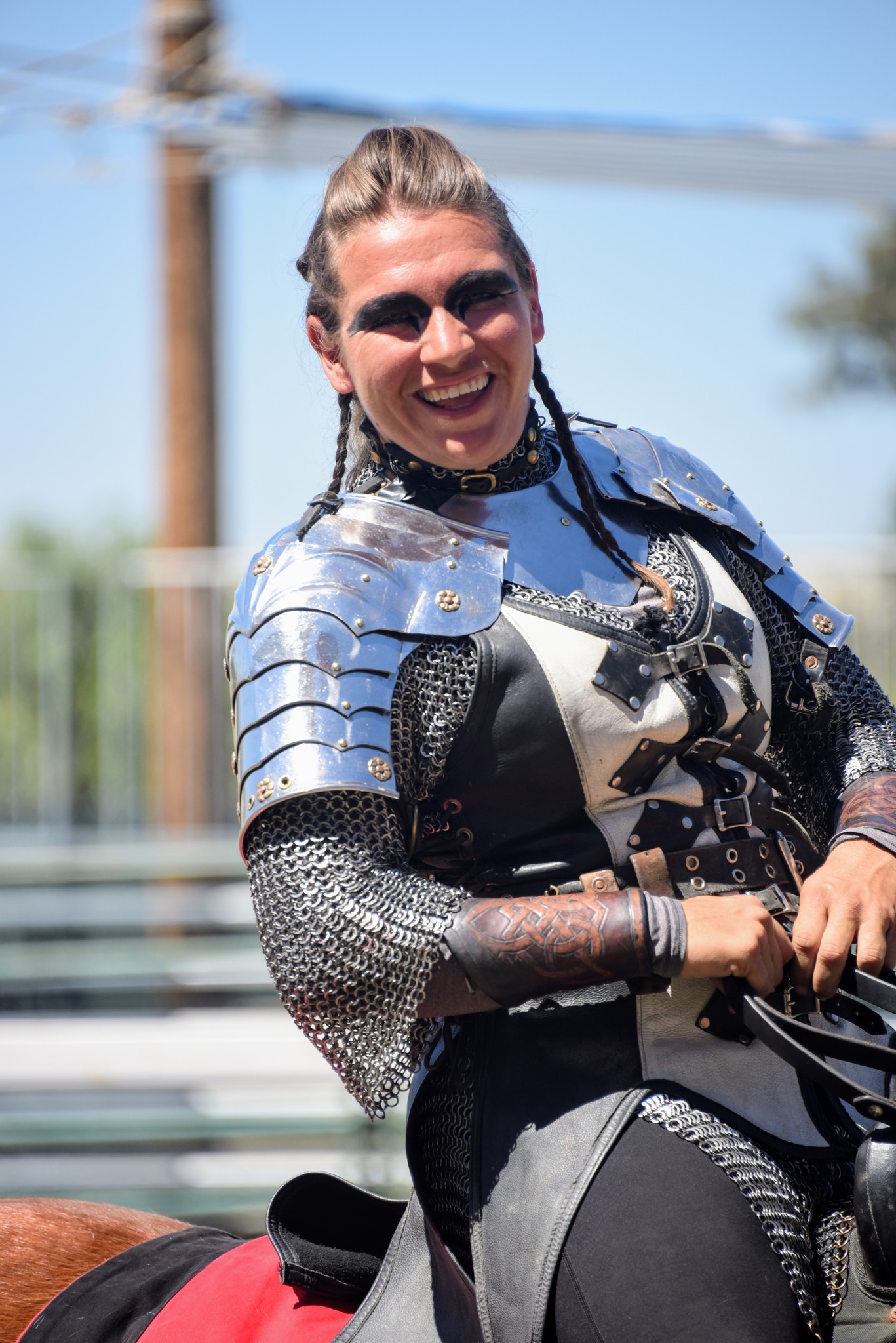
[0,1193,896,1343]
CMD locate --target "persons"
[223,125,896,1343]
